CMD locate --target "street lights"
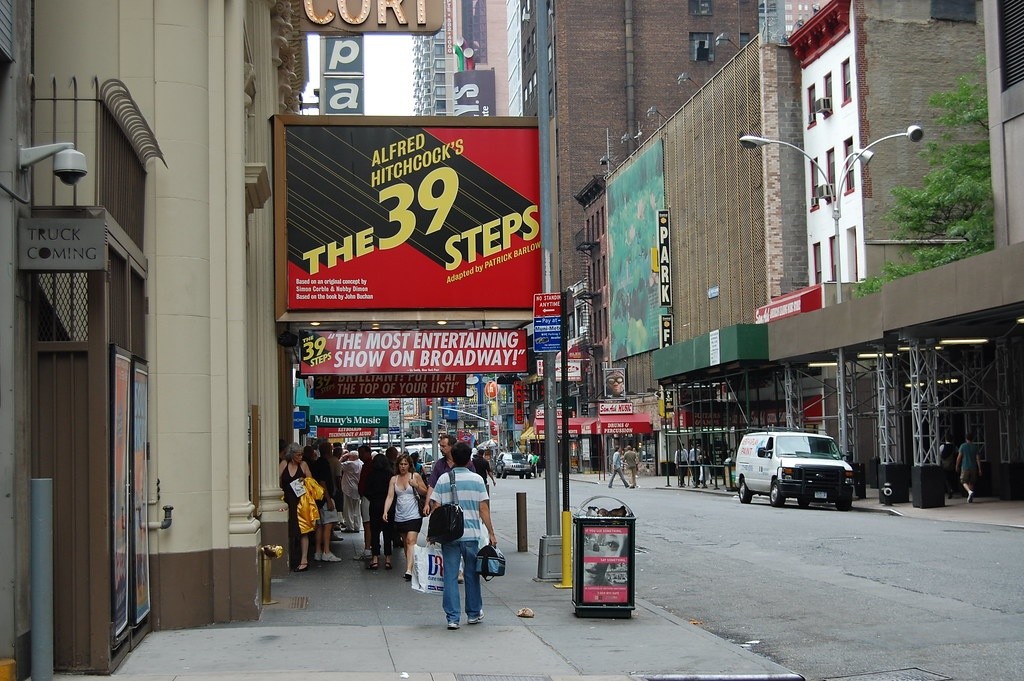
[739,125,926,459]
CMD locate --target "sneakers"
[448,623,459,628]
[314,552,322,560]
[322,551,341,562]
[354,553,372,560]
[468,608,484,623]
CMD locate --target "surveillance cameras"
[53,148,88,185]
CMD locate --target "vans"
[405,445,445,478]
[734,431,855,511]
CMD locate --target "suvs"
[496,453,533,479]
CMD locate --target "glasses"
[296,453,304,456]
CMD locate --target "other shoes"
[967,491,974,503]
[404,573,412,579]
[330,532,344,541]
[341,528,359,533]
[948,491,954,499]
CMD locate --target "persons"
[527,451,538,479]
[674,441,712,489]
[423,434,477,516]
[279,439,497,570]
[956,433,982,503]
[936,434,959,498]
[607,446,639,488]
[279,442,312,572]
[383,453,428,580]
[429,442,497,629]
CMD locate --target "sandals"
[371,560,379,570]
[384,562,392,569]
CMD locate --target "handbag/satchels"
[426,503,464,545]
[411,541,444,594]
[418,495,431,518]
[475,541,506,583]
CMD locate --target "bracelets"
[416,484,419,489]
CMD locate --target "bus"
[345,438,442,449]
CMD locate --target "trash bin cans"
[571,496,636,619]
[724,457,737,490]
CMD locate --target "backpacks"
[941,442,955,461]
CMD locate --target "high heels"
[294,563,308,573]
